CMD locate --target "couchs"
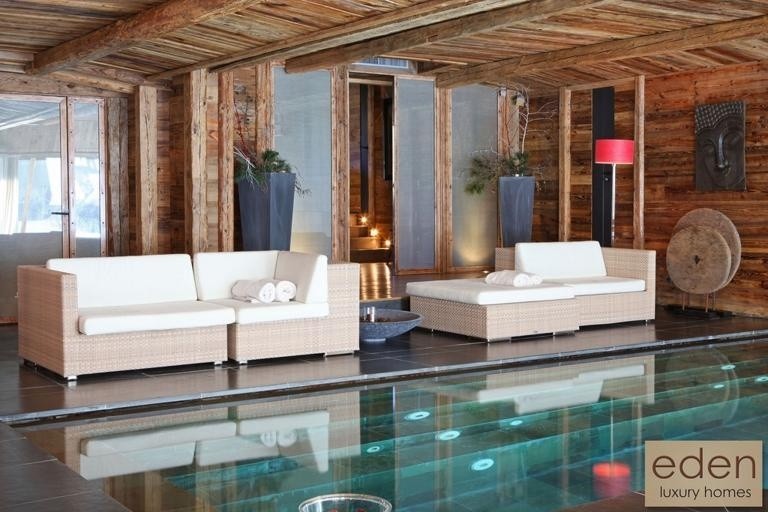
[15,248,362,389]
[405,243,660,345]
[32,390,365,474]
[411,353,659,417]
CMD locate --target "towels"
[261,430,298,450]
[484,269,541,289]
[229,275,298,305]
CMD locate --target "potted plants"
[463,147,562,246]
[231,139,312,250]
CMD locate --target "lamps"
[592,391,632,502]
[589,135,637,250]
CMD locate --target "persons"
[693,102,748,190]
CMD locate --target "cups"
[299,494,392,512]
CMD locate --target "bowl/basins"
[360,307,423,342]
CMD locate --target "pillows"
[513,239,607,282]
[517,380,602,419]
